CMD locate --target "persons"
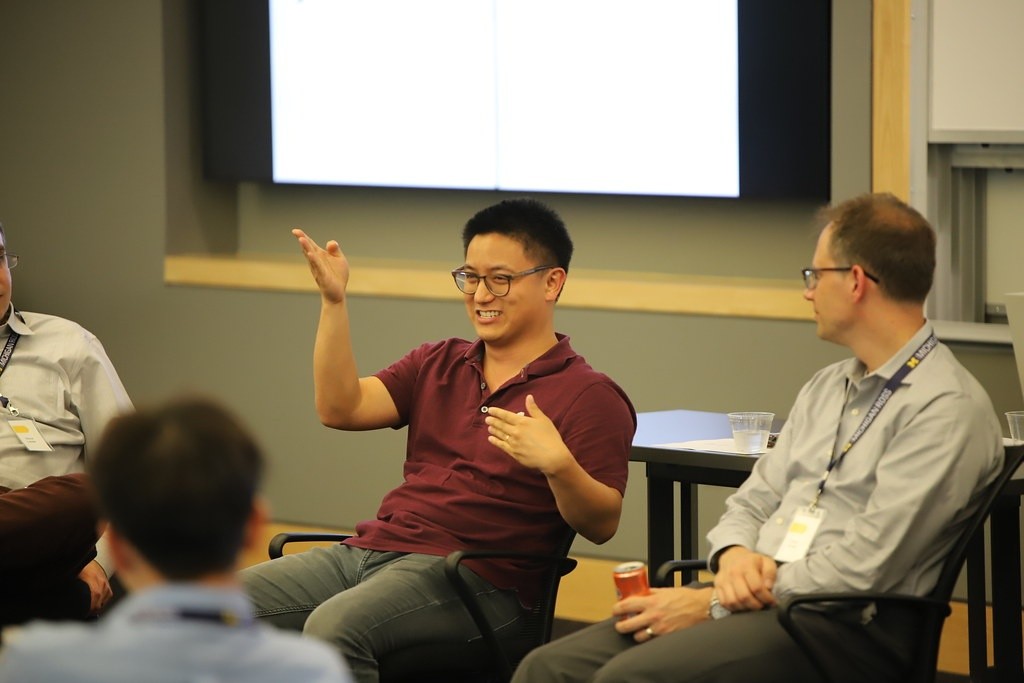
[1,225,140,626]
[513,193,1002,682]
[0,396,351,680]
[235,199,637,682]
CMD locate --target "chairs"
[268,532,579,682]
[656,443,1024,683]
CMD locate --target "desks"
[626,432,1023,683]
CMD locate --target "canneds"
[614,561,651,600]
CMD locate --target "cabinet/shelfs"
[909,1,1024,346]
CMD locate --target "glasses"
[802,266,880,290]
[0,250,18,270]
[452,265,559,298]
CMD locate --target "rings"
[646,628,654,637]
[506,435,509,441]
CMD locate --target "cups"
[728,411,775,453]
[1004,411,1024,446]
